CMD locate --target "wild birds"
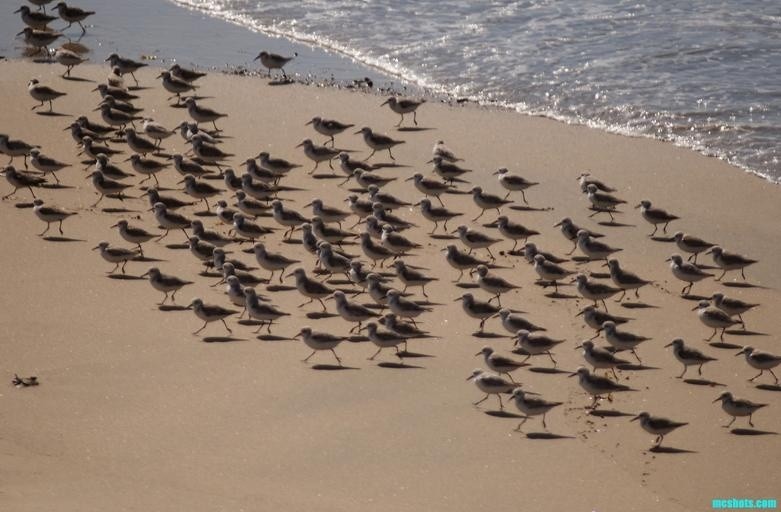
[16,27,63,57]
[0,48,781,451]
[14,5,60,33]
[51,2,96,34]
[27,0,52,15]
